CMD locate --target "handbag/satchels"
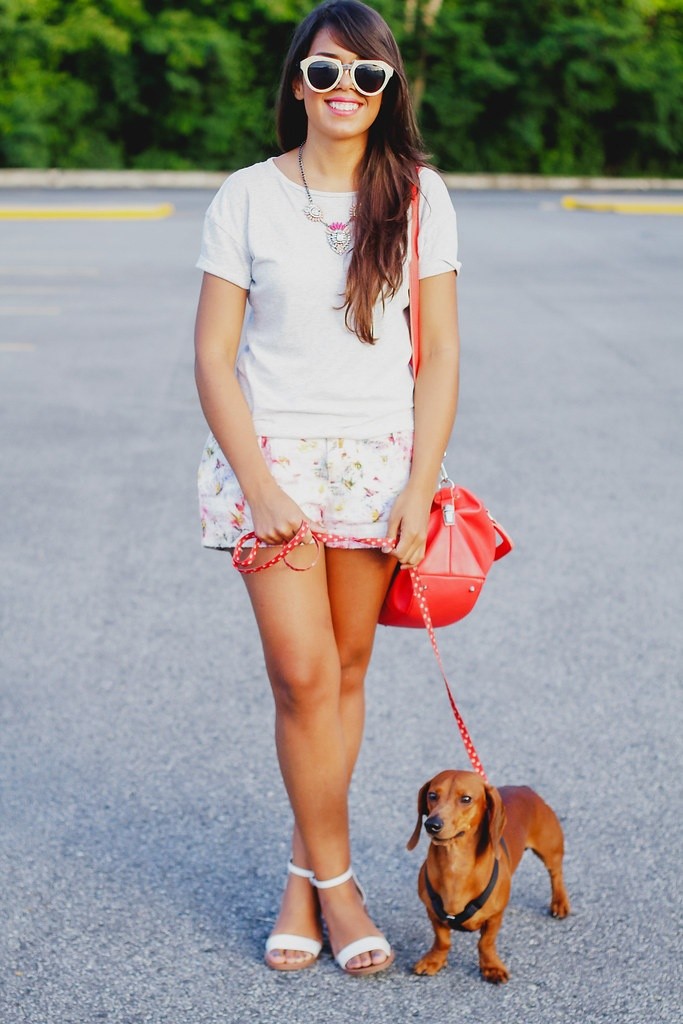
[379,481,512,630]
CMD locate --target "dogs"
[407,769,570,981]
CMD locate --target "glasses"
[299,58,393,95]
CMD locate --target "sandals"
[264,863,324,973]
[304,870,394,981]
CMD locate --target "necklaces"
[299,140,358,255]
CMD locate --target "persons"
[191,1,460,976]
